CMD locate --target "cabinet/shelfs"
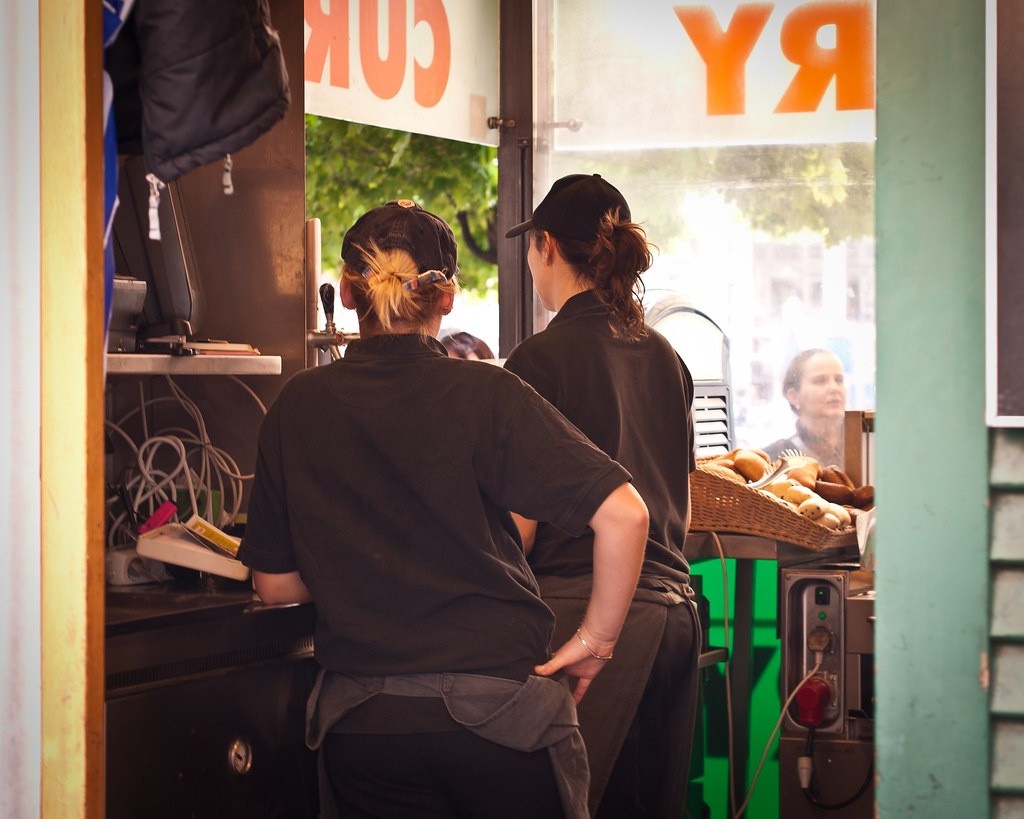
[103,653,318,819]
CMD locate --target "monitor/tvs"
[111,156,208,354]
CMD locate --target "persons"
[760,347,847,476]
[237,201,649,819]
[442,332,494,360]
[504,175,716,819]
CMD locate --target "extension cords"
[107,547,173,585]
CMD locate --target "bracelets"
[576,629,613,660]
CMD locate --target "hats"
[341,200,457,294]
[505,172,631,266]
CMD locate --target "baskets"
[689,454,860,551]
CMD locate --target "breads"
[699,448,876,532]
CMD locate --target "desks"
[683,530,822,819]
